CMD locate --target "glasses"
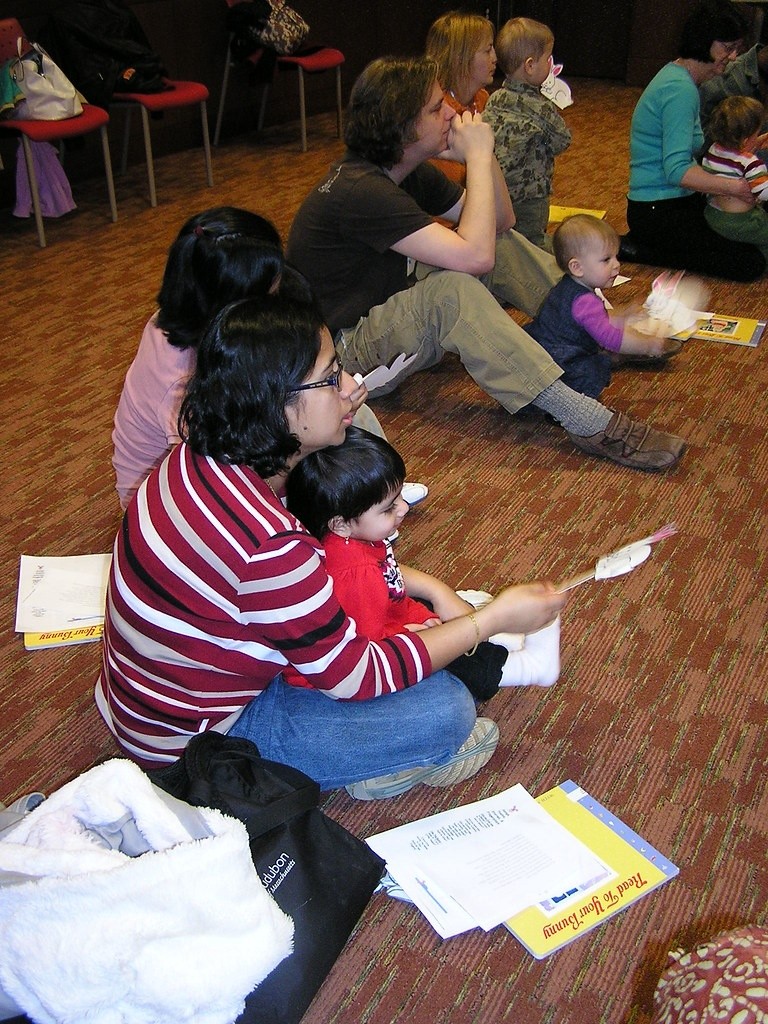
[721,41,742,55]
[286,351,344,394]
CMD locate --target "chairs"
[59,80,214,209]
[0,18,119,248]
[214,31,346,152]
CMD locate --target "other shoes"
[400,481,429,506]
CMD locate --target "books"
[15,554,110,649]
[632,307,767,348]
[499,779,677,960]
[550,205,608,225]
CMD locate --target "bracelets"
[265,478,279,498]
[464,615,479,656]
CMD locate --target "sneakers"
[568,406,686,471]
[344,716,499,801]
[608,337,684,363]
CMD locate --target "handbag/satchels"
[112,39,174,94]
[247,0,311,58]
[0,730,388,1024]
[4,36,83,122]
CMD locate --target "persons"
[483,17,571,250]
[108,206,429,519]
[280,427,507,703]
[285,54,685,471]
[93,297,571,802]
[395,8,502,232]
[699,96,767,246]
[624,0,768,283]
[519,212,668,397]
[697,5,768,167]
